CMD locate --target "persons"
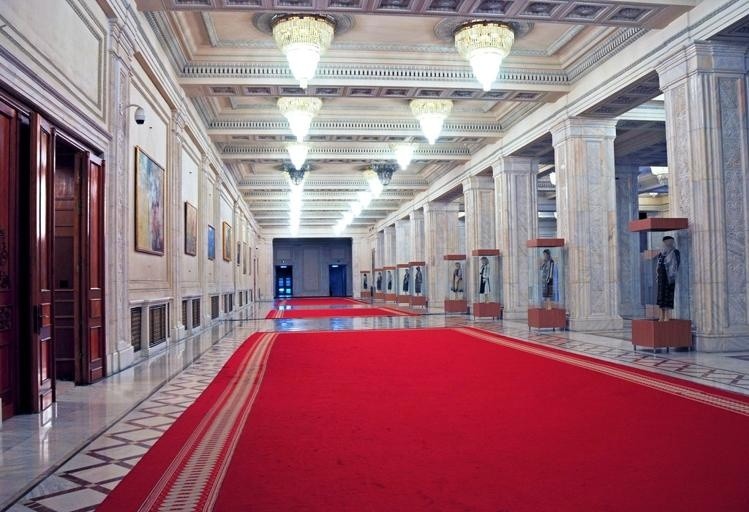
[403,269,409,292]
[363,274,367,289]
[388,271,392,290]
[376,271,382,290]
[656,236,680,322]
[415,267,422,293]
[539,249,554,310]
[479,257,490,304]
[452,262,463,291]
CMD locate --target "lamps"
[394,16,534,172]
[251,11,355,173]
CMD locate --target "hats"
[663,236,674,240]
[481,257,486,259]
[544,249,550,255]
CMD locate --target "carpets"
[96,325,748,512]
[267,305,421,320]
[273,295,371,306]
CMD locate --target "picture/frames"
[186,200,198,257]
[223,222,233,261]
[207,224,216,261]
[135,144,167,256]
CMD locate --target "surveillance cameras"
[134,106,146,124]
